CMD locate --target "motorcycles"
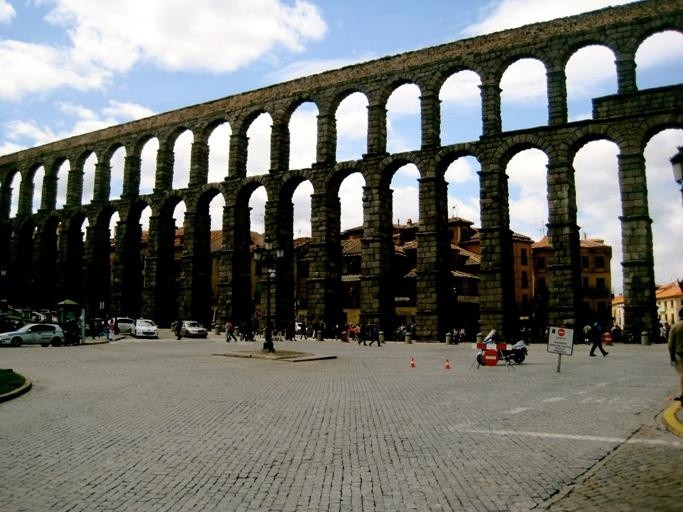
[476,329,528,366]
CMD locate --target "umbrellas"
[57,299,78,306]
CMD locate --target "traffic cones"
[444,359,450,370]
[410,357,416,369]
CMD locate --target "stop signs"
[484,349,498,365]
[603,332,613,345]
[558,328,565,336]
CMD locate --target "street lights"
[253,236,284,353]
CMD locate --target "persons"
[451,327,466,345]
[225,320,258,343]
[583,323,592,345]
[611,324,622,341]
[666,309,682,405]
[312,319,325,339]
[335,320,384,347]
[104,313,114,335]
[492,331,504,344]
[398,321,416,341]
[587,322,608,358]
[300,319,307,339]
[64,320,80,347]
[174,318,183,340]
[653,319,663,344]
[285,319,296,341]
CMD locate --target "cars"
[130,318,160,339]
[0,322,65,347]
[171,320,209,337]
[108,317,134,333]
[397,323,416,338]
[93,314,110,326]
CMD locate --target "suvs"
[295,322,307,335]
